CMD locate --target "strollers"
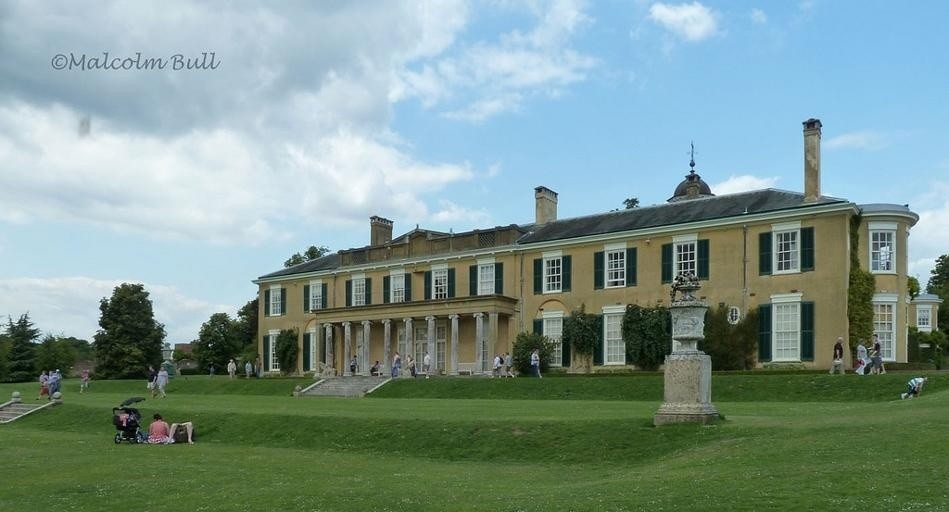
[111,395,148,446]
[855,343,875,376]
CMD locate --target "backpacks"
[499,356,504,364]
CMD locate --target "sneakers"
[900,393,905,400]
[880,370,887,375]
[425,376,429,379]
[867,371,873,376]
[149,393,169,400]
[874,370,879,375]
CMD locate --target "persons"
[491,353,504,379]
[388,351,401,378]
[78,366,89,394]
[423,350,432,380]
[146,364,157,399]
[35,370,63,402]
[856,338,868,376]
[369,359,383,376]
[504,351,515,379]
[350,354,359,376]
[155,366,169,399]
[900,374,928,401]
[245,359,252,380]
[530,348,543,378]
[406,355,418,378]
[867,333,887,376]
[147,413,169,443]
[228,359,238,380]
[826,336,845,376]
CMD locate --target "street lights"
[207,343,213,373]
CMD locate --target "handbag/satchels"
[831,358,841,366]
